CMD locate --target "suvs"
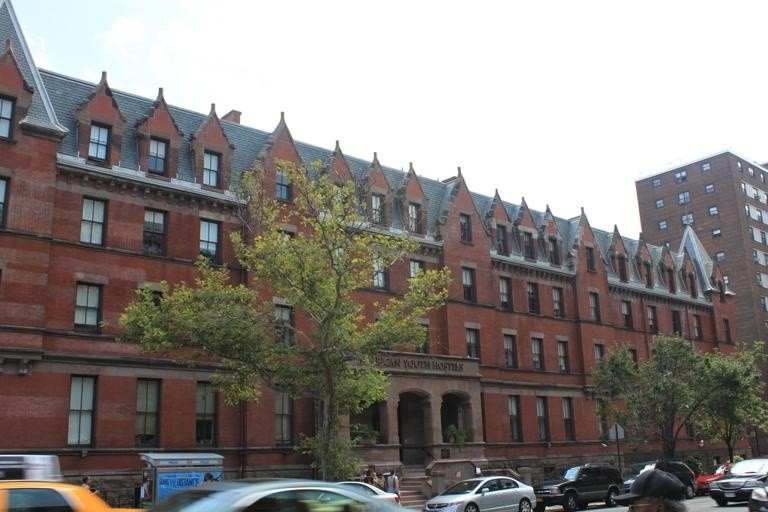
[619,459,694,506]
[532,462,624,512]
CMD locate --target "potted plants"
[445,424,469,447]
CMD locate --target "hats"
[609,467,686,508]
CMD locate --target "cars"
[422,476,536,512]
[692,457,768,512]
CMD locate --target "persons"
[363,464,399,495]
[81,476,100,494]
[613,470,688,512]
